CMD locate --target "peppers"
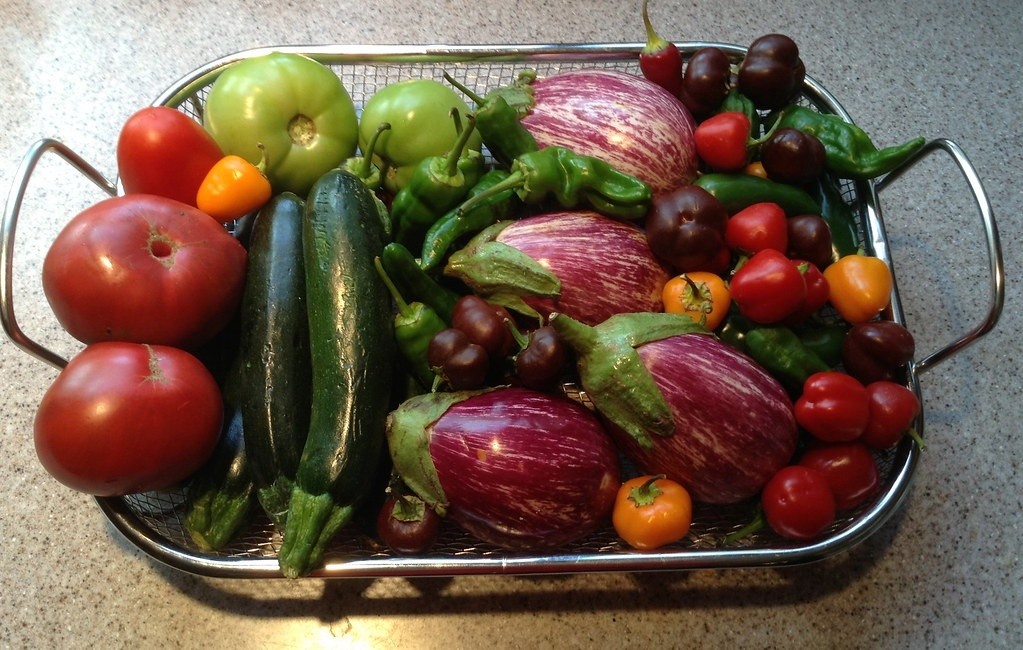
[195,0,925,554]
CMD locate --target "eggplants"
[546,309,798,506]
[441,210,620,325]
[479,68,697,199]
[384,384,621,552]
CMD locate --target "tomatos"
[41,193,246,348]
[116,106,224,211]
[359,77,483,195]
[32,340,222,496]
[202,53,359,196]
